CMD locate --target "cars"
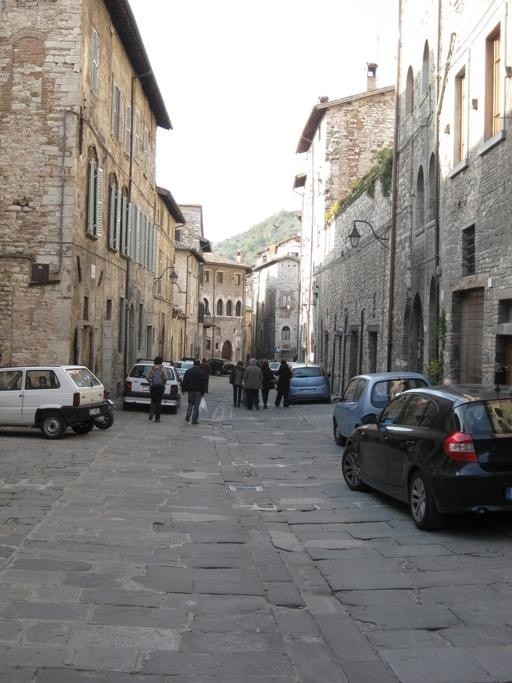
[121,357,237,415]
[335,381,511,527]
[329,372,434,447]
[262,360,335,405]
[0,364,115,439]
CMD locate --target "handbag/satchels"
[229,373,235,383]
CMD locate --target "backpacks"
[151,365,164,387]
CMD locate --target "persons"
[181,358,210,423]
[229,358,292,410]
[146,356,168,423]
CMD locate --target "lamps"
[347,218,390,251]
[154,265,178,286]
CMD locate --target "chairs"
[26,375,49,388]
